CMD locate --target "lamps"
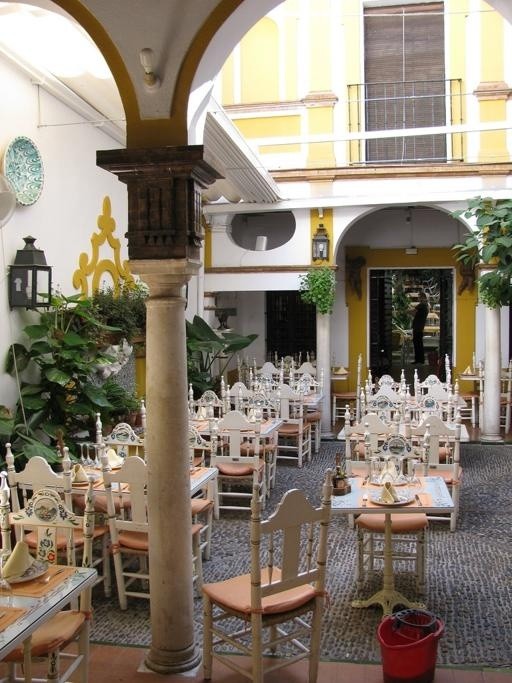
[7,233,53,311]
[405,247,419,256]
[312,224,331,263]
[139,46,162,92]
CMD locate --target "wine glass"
[188,400,207,430]
[0,548,12,618]
[251,377,275,399]
[392,383,412,402]
[368,458,420,488]
[76,440,104,484]
[399,409,425,431]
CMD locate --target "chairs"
[0,350,512,683]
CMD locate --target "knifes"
[414,494,422,505]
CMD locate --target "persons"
[398,293,430,364]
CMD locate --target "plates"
[370,490,416,505]
[96,460,122,471]
[71,471,102,484]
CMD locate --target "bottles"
[334,465,349,489]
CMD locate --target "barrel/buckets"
[376,610,444,682]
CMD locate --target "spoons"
[33,566,66,584]
[0,550,49,583]
